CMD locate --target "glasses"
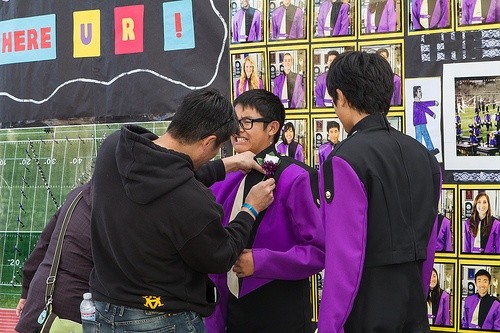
[235,117,269,130]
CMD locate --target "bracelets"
[241,204,257,217]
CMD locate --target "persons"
[236,56,263,97]
[435,193,500,331]
[318,51,442,333]
[274,53,304,108]
[14,180,95,333]
[277,122,304,163]
[207,90,325,333]
[82,88,275,333]
[318,120,342,167]
[365,0,395,34]
[231,0,262,42]
[412,0,450,29]
[456,100,500,156]
[462,0,500,24]
[375,48,401,106]
[271,0,304,40]
[427,268,450,325]
[314,51,339,108]
[317,0,349,36]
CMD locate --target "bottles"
[80,292,95,321]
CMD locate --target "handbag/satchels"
[39,309,85,333]
